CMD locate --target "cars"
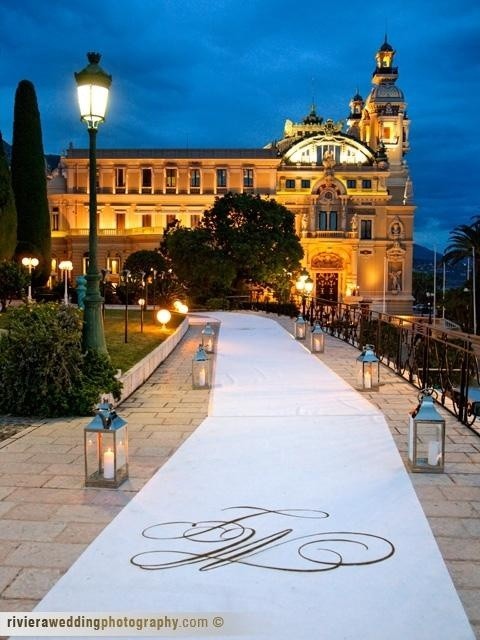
[412,304,425,311]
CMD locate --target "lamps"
[157,299,189,330]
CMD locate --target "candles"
[299,328,302,337]
[199,368,205,386]
[365,371,371,388]
[428,441,439,465]
[316,340,320,351]
[208,339,212,351]
[103,449,114,479]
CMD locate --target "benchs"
[222,296,250,310]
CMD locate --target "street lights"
[296,267,314,320]
[22,257,40,305]
[139,269,167,333]
[464,287,471,331]
[59,260,74,306]
[426,292,435,324]
[73,49,114,371]
[122,269,131,343]
[101,267,111,321]
